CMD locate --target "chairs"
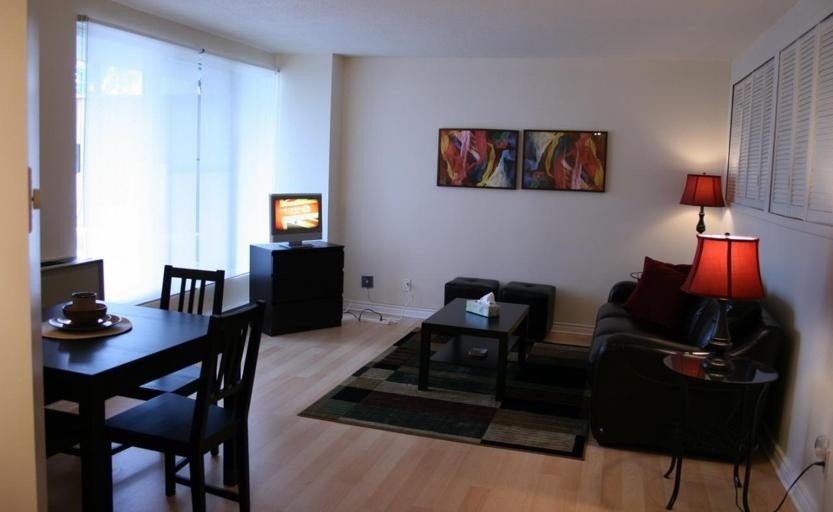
[116,265,224,458]
[106,299,267,511]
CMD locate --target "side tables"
[661,350,780,512]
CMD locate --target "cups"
[70,291,95,309]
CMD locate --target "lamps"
[680,233,766,374]
[679,172,725,233]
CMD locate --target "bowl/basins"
[61,303,107,320]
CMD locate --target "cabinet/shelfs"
[249,240,344,338]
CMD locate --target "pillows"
[619,255,704,333]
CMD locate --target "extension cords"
[377,318,390,324]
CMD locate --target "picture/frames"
[521,129,609,192]
[437,127,519,190]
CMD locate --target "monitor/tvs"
[269,193,322,248]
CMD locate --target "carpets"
[296,323,591,460]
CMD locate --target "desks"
[42,298,210,512]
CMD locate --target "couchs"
[588,279,791,462]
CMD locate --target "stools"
[500,281,557,340]
[443,276,500,306]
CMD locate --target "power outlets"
[400,279,411,293]
[361,275,374,288]
[821,449,832,479]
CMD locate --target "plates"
[47,313,120,331]
